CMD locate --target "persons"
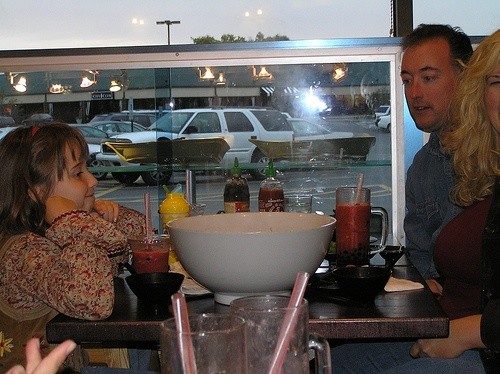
[398,24,474,284]
[0,120,158,374]
[308,28,500,374]
[5,337,77,374]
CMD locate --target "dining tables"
[45,233,452,346]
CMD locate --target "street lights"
[156,19,180,105]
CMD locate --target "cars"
[0,116,17,128]
[373,103,390,132]
[22,113,54,127]
[66,123,150,177]
[287,117,354,139]
[95,107,296,183]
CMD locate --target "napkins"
[383,272,426,294]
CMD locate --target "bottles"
[159,185,191,264]
[258,163,284,212]
[224,158,251,213]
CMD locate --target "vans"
[89,114,156,139]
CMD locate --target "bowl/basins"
[167,212,337,305]
[332,267,391,298]
[325,245,406,267]
[125,272,185,306]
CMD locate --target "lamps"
[251,66,272,83]
[79,70,103,88]
[213,72,227,85]
[44,71,65,94]
[109,69,129,92]
[332,63,349,81]
[197,67,216,83]
[5,72,28,93]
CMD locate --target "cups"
[126,234,171,273]
[191,203,206,217]
[336,187,388,266]
[284,194,312,212]
[230,295,332,374]
[160,313,247,374]
